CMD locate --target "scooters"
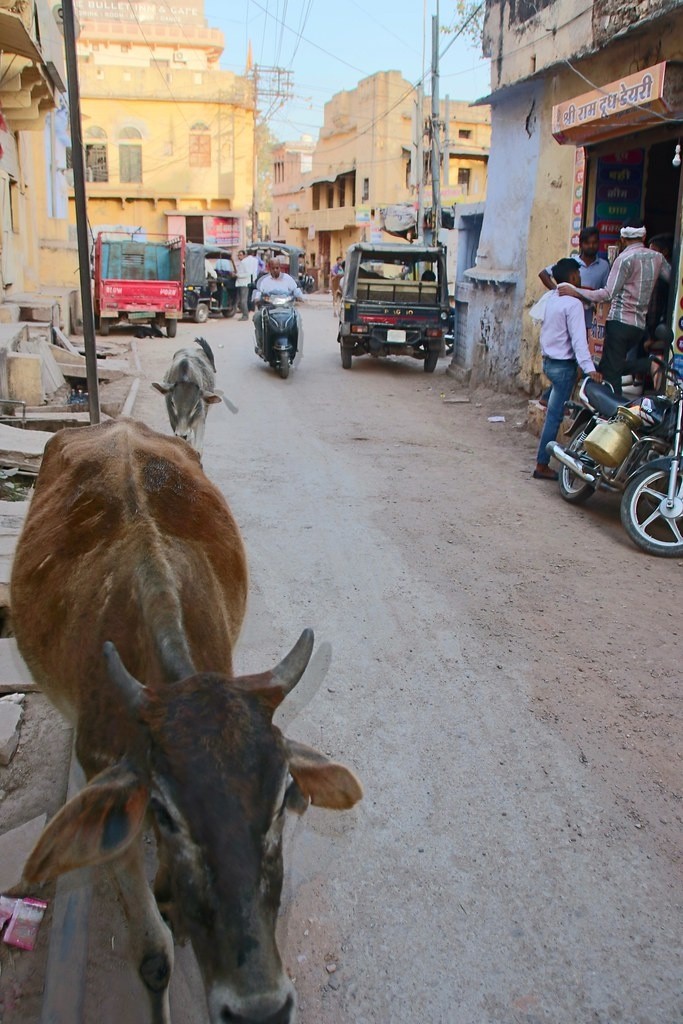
[247,285,299,379]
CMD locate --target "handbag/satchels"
[247,274,257,289]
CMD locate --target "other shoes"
[238,315,249,321]
[650,354,665,391]
[538,397,570,415]
[533,463,559,480]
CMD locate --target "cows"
[152,336,222,459]
[0,417,365,1024]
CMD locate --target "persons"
[230,248,265,321]
[621,232,675,395]
[538,226,611,415]
[420,269,455,329]
[533,257,603,481]
[253,256,308,355]
[330,256,345,293]
[558,217,672,396]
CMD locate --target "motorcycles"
[545,323,683,559]
[337,242,448,372]
[406,281,489,357]
[185,227,314,324]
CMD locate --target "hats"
[619,225,647,238]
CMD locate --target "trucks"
[90,230,185,338]
